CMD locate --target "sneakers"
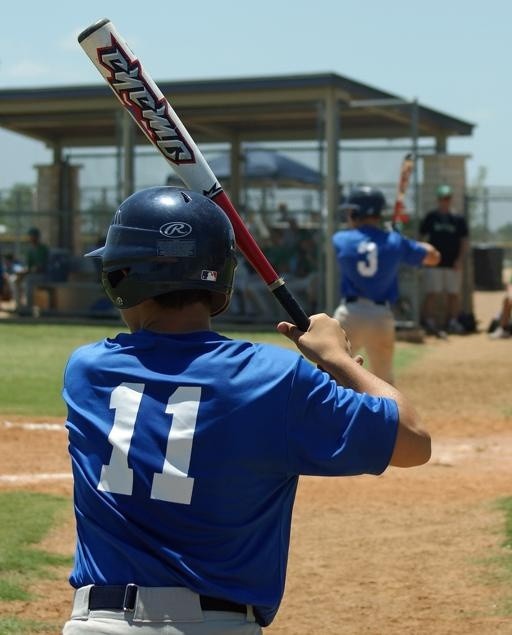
[426,322,464,338]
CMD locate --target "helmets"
[84,186,239,317]
[338,186,386,218]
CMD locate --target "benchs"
[20,279,309,322]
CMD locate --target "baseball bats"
[78,16,310,331]
[392,153,416,230]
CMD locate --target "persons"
[228,203,326,321]
[417,185,468,335]
[0,227,53,316]
[60,186,432,635]
[332,182,440,385]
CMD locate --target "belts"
[89,586,258,616]
[346,296,386,306]
